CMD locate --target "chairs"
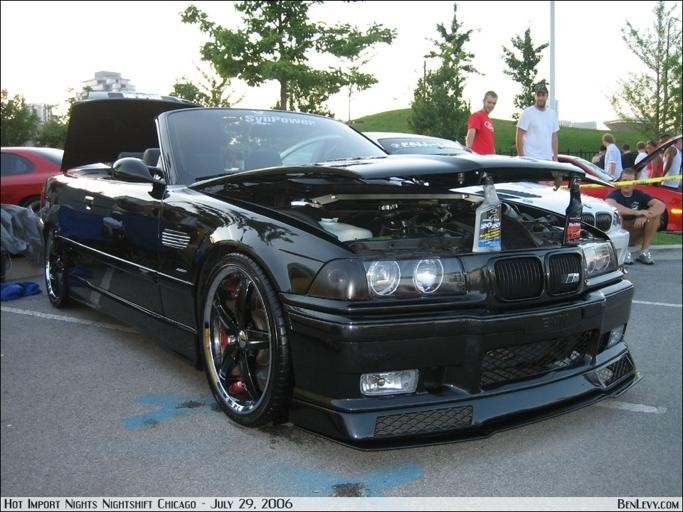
[118,148,162,176]
[244,150,283,171]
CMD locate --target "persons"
[605,167,666,265]
[515,84,561,161]
[466,90,498,155]
[603,132,624,181]
[592,135,683,192]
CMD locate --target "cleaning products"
[472,170,502,254]
[562,174,582,246]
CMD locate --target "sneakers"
[636,249,656,265]
[624,251,634,265]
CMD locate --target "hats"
[533,84,549,95]
